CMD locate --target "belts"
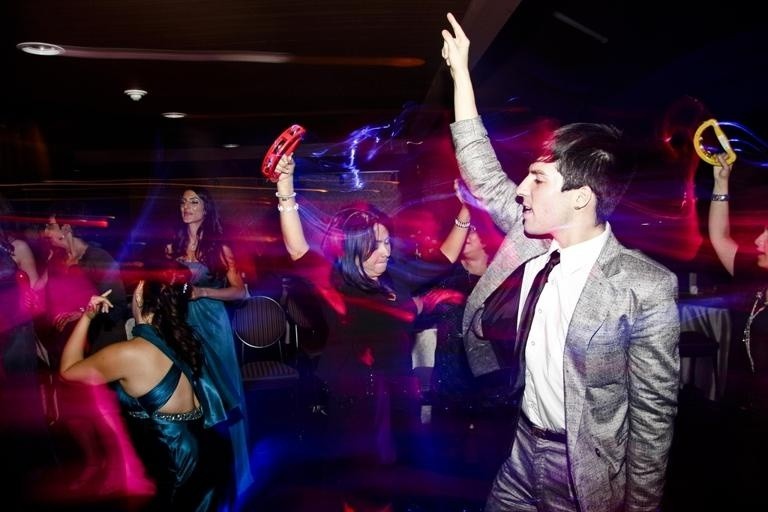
[519,411,565,443]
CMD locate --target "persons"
[272,151,481,511]
[705,152,768,416]
[411,208,508,511]
[442,10,686,509]
[1,186,256,511]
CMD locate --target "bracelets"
[276,191,297,198]
[710,193,730,202]
[454,217,471,228]
[456,216,472,221]
[277,203,300,212]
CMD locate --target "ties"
[504,253,559,399]
